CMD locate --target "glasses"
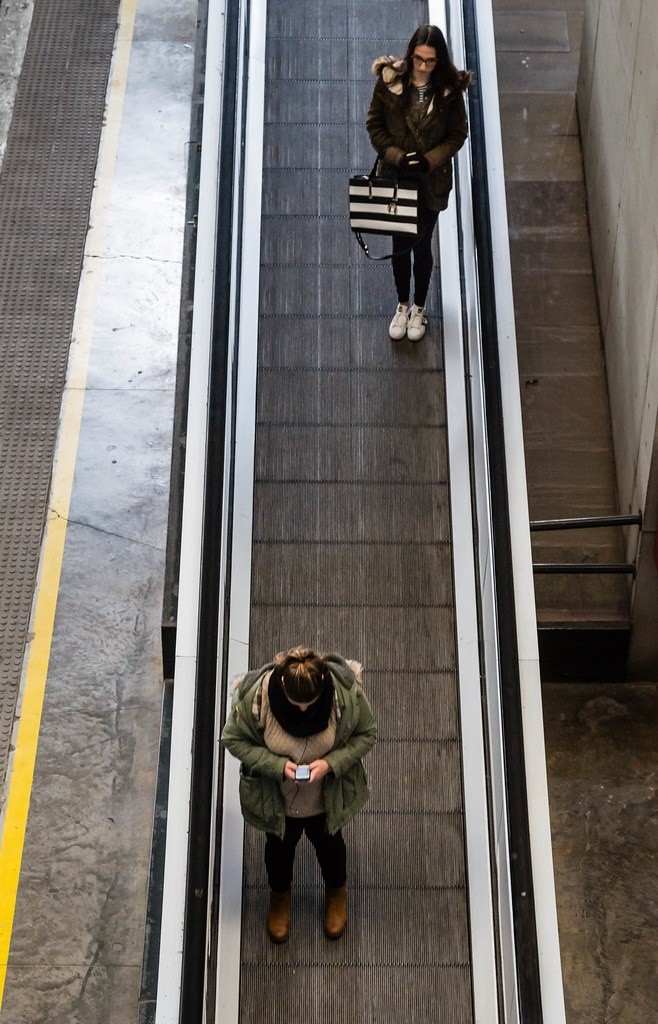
[411,52,438,67]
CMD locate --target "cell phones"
[295,764,310,780]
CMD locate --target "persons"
[366,23,469,340]
[221,645,377,944]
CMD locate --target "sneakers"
[407,303,426,340]
[388,303,410,341]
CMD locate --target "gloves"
[401,149,430,177]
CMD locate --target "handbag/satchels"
[348,153,420,238]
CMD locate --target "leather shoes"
[267,885,291,941]
[323,885,348,937]
